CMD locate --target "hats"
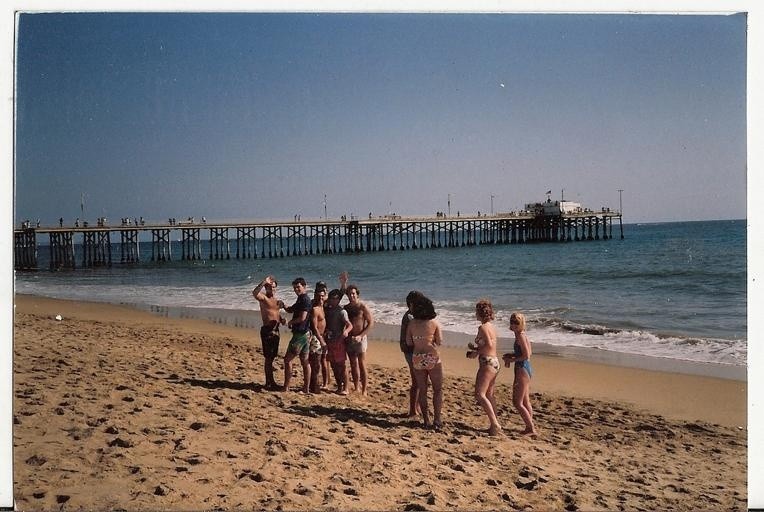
[316,281,326,288]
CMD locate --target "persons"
[406,298,443,429]
[253,271,372,397]
[466,300,502,436]
[400,290,424,418]
[502,312,538,437]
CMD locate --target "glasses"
[510,320,519,324]
